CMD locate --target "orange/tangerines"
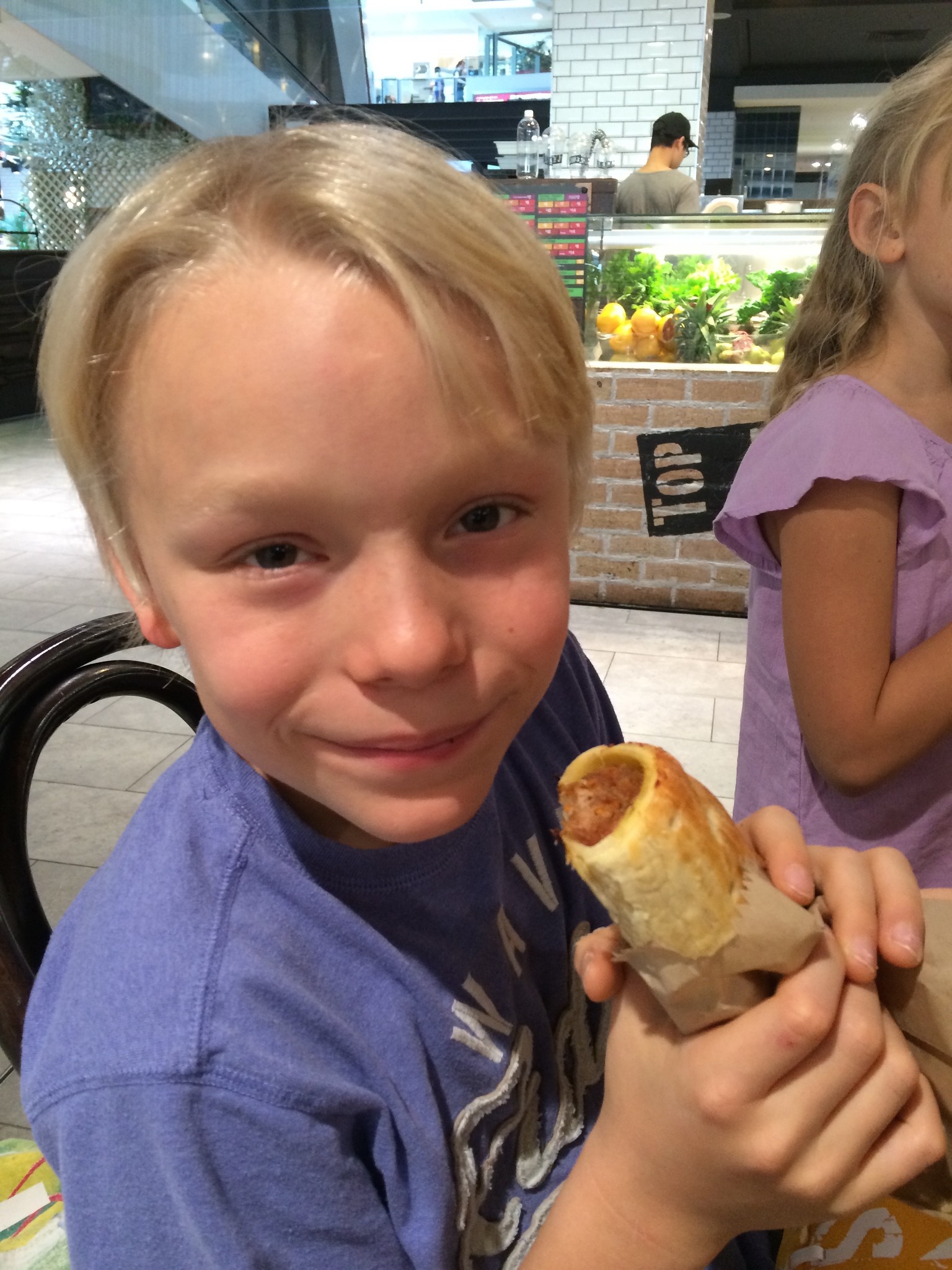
[596,303,674,362]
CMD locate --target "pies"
[554,742,750,955]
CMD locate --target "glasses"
[682,141,690,158]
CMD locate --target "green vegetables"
[602,250,817,331]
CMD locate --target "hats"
[652,111,699,149]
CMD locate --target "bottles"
[516,110,539,179]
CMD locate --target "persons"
[17,129,944,1268]
[708,38,947,897]
[452,59,468,101]
[611,112,702,219]
[433,66,447,102]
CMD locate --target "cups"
[542,126,566,177]
[567,131,591,179]
[593,138,618,179]
